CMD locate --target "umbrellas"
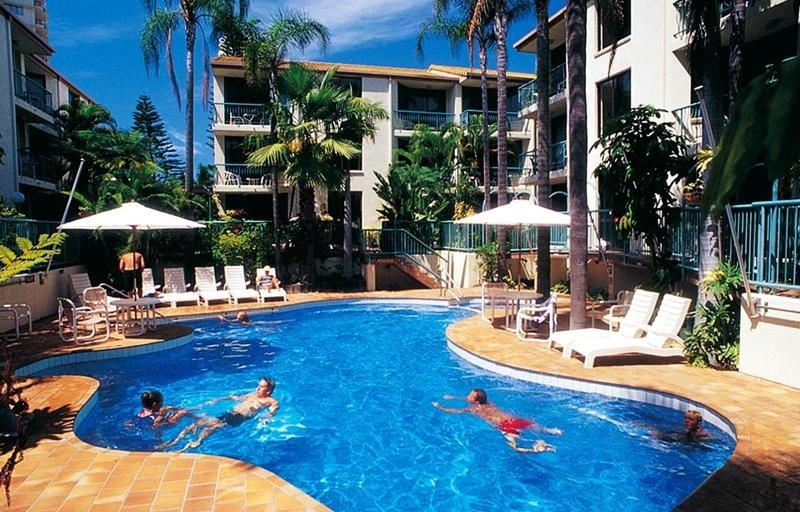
[452,196,571,314]
[55,199,207,321]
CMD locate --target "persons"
[431,390,564,453]
[154,377,280,448]
[257,265,281,294]
[121,391,206,434]
[631,408,712,446]
[119,246,144,297]
[216,310,295,326]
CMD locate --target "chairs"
[481,280,703,374]
[0,257,287,349]
